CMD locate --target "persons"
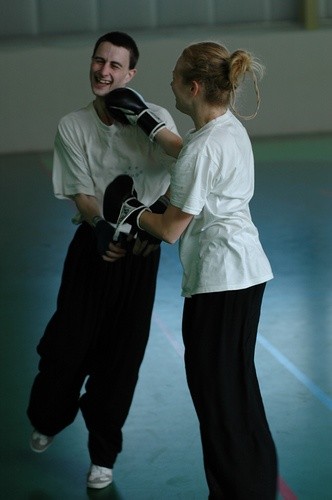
[103,41,279,500]
[26,31,181,489]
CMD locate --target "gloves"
[102,87,167,144]
[102,173,152,230]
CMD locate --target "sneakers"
[30,429,54,453]
[86,463,113,490]
